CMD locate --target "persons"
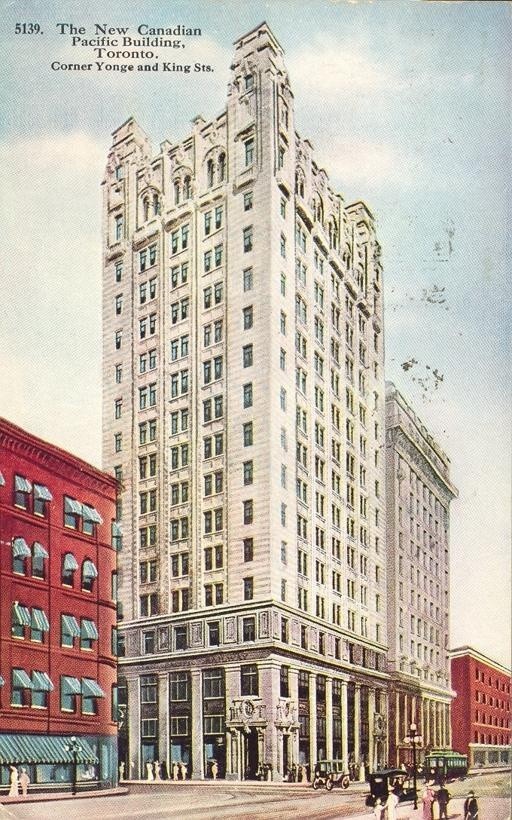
[255,761,273,781]
[384,762,424,781]
[286,761,310,783]
[373,786,400,820]
[172,760,190,780]
[421,782,450,820]
[119,760,127,781]
[349,764,357,781]
[146,758,162,783]
[463,790,479,820]
[9,765,30,797]
[210,762,218,779]
